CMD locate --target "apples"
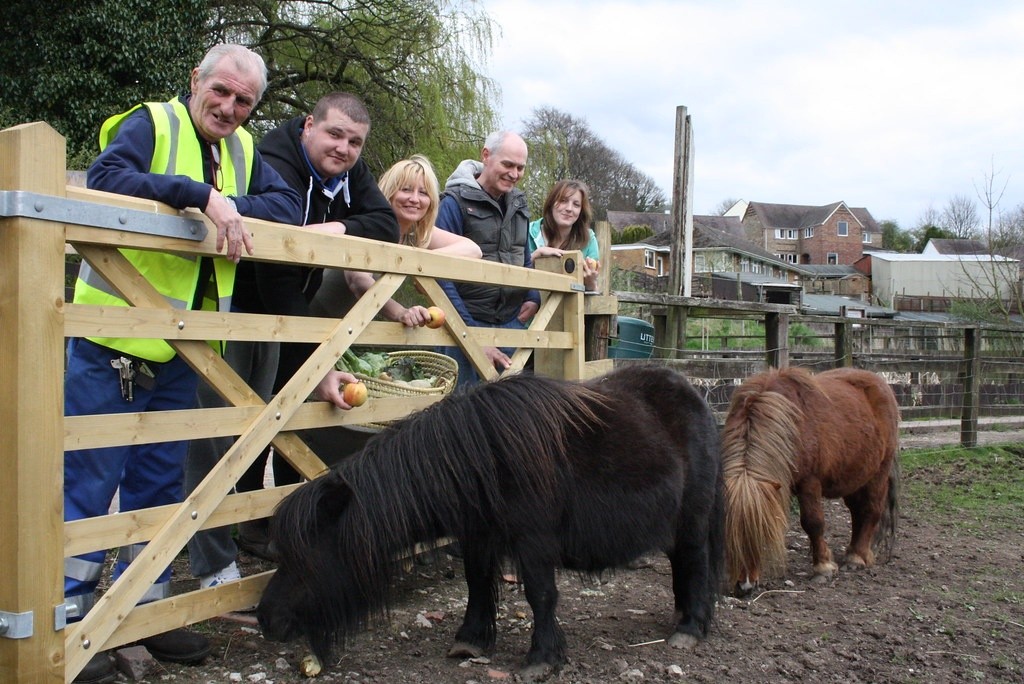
[424,306,445,329]
[344,382,367,407]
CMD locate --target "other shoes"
[236,535,281,563]
[444,541,465,559]
[400,552,435,574]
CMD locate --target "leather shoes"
[68,650,121,684]
[134,629,212,662]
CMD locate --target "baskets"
[341,350,458,434]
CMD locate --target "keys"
[110,356,137,402]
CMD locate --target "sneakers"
[199,560,259,611]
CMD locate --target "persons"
[529,178,602,293]
[187,91,401,589]
[62,43,303,683]
[432,129,544,392]
[273,153,485,488]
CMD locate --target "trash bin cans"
[608,316,657,360]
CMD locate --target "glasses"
[207,142,223,192]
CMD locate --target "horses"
[713,367,900,596]
[255,364,726,683]
